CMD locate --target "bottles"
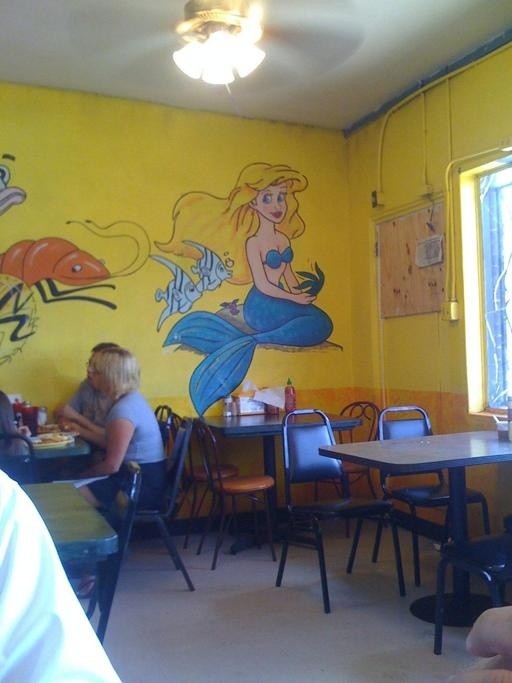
[285,375,297,415]
[224,393,234,416]
[508,396,512,442]
[12,397,49,437]
[271,405,279,415]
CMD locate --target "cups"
[497,421,507,438]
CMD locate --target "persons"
[0,469,124,683]
[0,389,25,488]
[444,604,512,683]
[53,341,123,453]
[58,346,170,598]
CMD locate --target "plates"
[38,432,81,438]
[30,435,75,449]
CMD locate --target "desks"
[9,478,123,569]
[312,427,510,477]
[190,413,364,440]
[4,402,95,460]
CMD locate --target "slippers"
[76,573,95,600]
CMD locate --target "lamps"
[172,0,266,85]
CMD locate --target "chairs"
[272,407,410,615]
[310,401,392,540]
[108,416,199,593]
[145,404,171,451]
[431,514,510,656]
[2,429,39,489]
[369,404,491,589]
[169,412,243,555]
[189,417,285,572]
[79,459,147,650]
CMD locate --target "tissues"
[233,381,285,417]
